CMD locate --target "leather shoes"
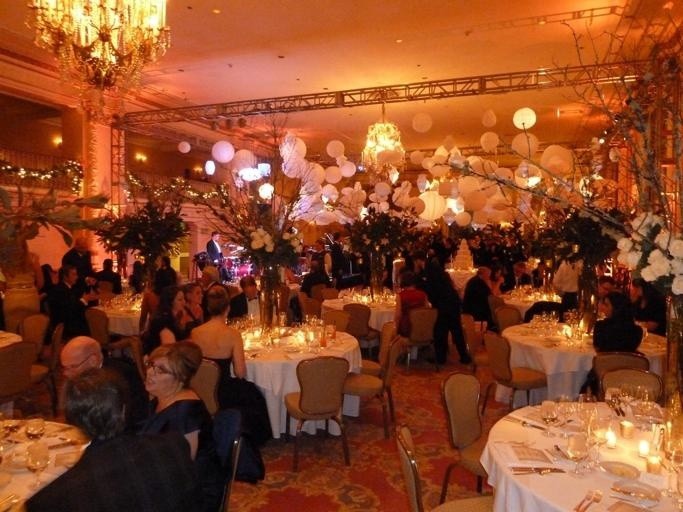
[428,356,445,364]
[460,358,469,363]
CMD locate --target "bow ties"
[248,297,257,302]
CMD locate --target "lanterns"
[176,108,575,228]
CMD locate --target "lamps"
[361,119,406,173]
[205,115,248,133]
[24,1,174,123]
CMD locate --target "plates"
[6,453,51,473]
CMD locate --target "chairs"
[481,332,547,415]
[295,282,439,364]
[458,303,523,377]
[282,355,352,470]
[434,372,489,504]
[392,422,496,510]
[188,405,246,510]
[339,320,409,440]
[590,352,664,405]
[1,286,148,425]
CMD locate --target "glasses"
[145,360,174,378]
[59,359,88,374]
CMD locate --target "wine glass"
[2,409,23,449]
[229,313,336,352]
[528,310,578,343]
[24,442,51,490]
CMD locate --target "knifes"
[47,438,76,450]
[504,414,545,431]
[510,466,564,476]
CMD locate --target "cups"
[24,413,45,441]
[539,383,683,506]
[109,292,142,315]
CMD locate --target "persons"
[142,342,224,511]
[59,336,146,428]
[36,216,664,397]
[188,284,272,485]
[22,357,203,511]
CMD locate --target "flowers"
[425,17,682,317]
[180,109,327,277]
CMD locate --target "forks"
[572,487,602,512]
